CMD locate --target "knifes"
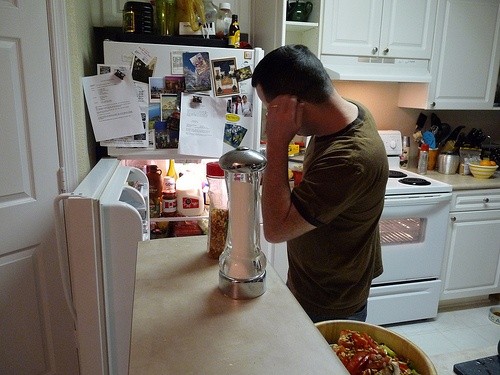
[465,128,487,148]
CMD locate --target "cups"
[418,146,439,170]
[155,0,176,37]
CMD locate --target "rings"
[271,104,280,107]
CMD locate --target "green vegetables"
[380,344,424,375]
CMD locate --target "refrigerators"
[51,39,264,375]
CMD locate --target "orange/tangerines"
[479,160,496,166]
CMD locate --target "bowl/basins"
[467,162,499,179]
[313,320,438,375]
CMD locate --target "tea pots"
[287,0,313,22]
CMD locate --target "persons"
[251,44,389,325]
[233,95,252,117]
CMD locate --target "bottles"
[123,1,154,35]
[417,143,429,175]
[438,150,460,175]
[227,15,241,48]
[145,158,204,218]
[400,136,410,169]
[205,161,229,259]
[218,146,268,301]
[215,3,232,41]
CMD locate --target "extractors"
[321,54,433,83]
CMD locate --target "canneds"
[160,191,177,217]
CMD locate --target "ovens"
[365,193,453,325]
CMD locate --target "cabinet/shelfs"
[398,0,500,111]
[251,0,325,60]
[438,188,500,310]
[301,0,438,59]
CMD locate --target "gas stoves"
[377,130,453,195]
